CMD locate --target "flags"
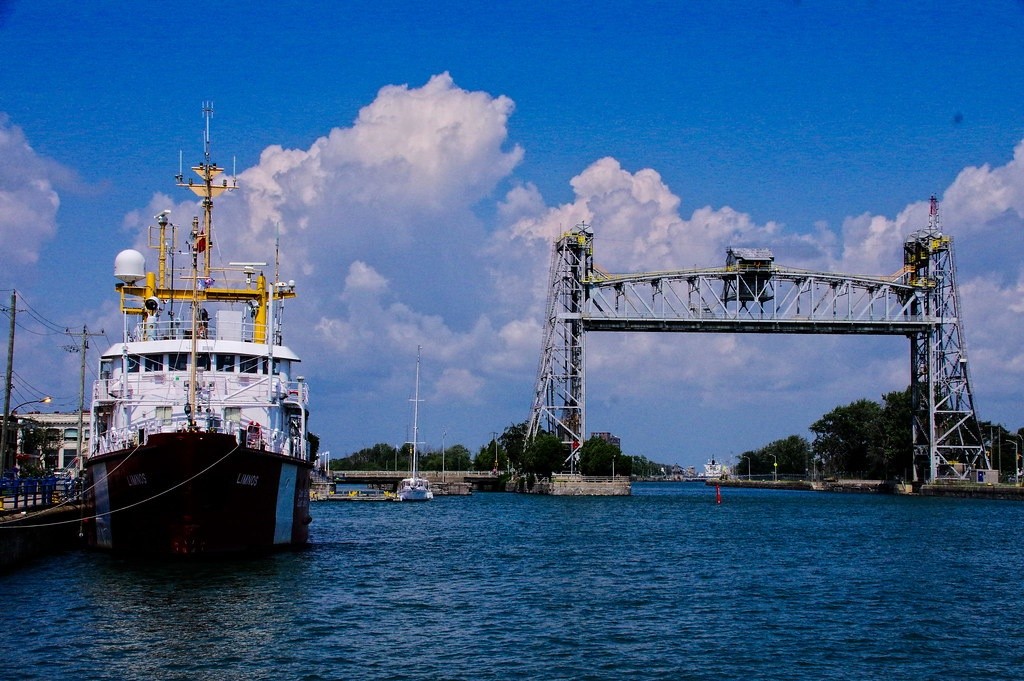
[192,225,206,253]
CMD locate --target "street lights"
[11,397,52,414]
[323,451,329,477]
[769,454,776,481]
[490,432,497,474]
[744,456,750,480]
[1006,440,1019,484]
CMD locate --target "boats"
[81,102,320,555]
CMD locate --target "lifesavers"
[246,420,263,440]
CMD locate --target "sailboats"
[397,344,435,501]
